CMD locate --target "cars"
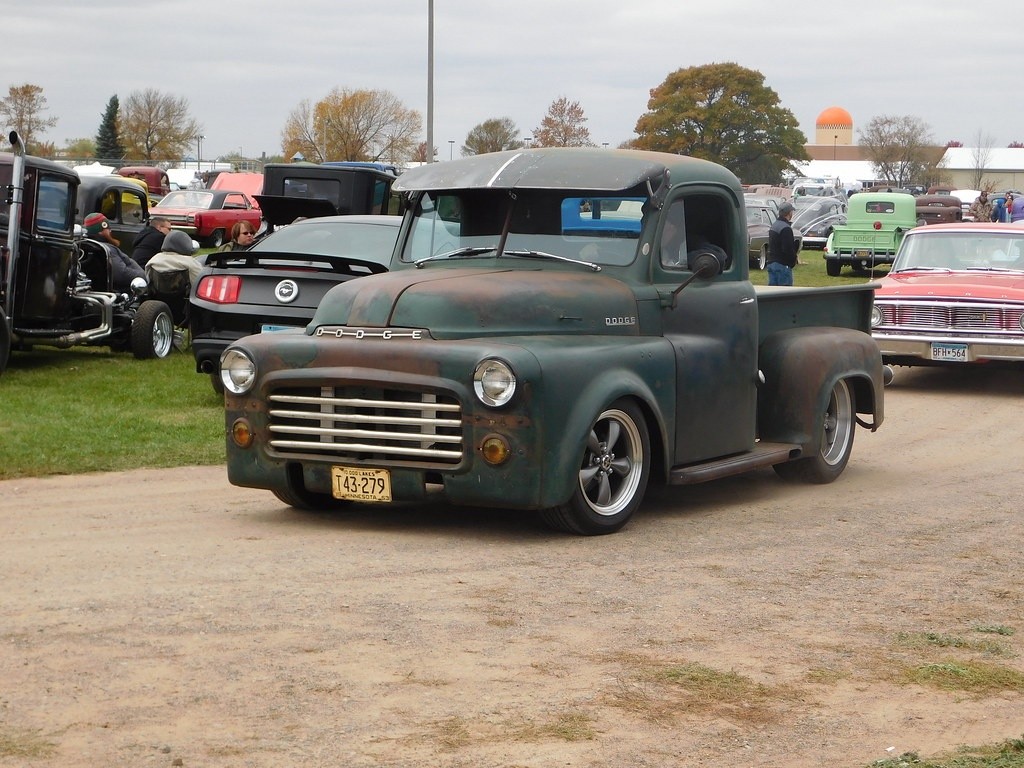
[188,216,460,397]
[866,222,1024,368]
[869,184,1024,225]
[36,161,405,260]
[740,178,850,270]
[0,131,175,361]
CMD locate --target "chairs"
[74,239,117,294]
[925,239,961,270]
[146,263,194,356]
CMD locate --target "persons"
[970,190,1014,224]
[765,202,798,286]
[80,212,256,309]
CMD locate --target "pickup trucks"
[217,146,896,537]
[822,192,927,277]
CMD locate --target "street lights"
[834,135,837,160]
[194,134,204,171]
[601,143,609,148]
[524,137,532,149]
[238,145,262,173]
[316,116,326,162]
[448,141,455,160]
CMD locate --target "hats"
[779,202,796,217]
[83,212,111,235]
[641,194,683,227]
[1007,195,1013,201]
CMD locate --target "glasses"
[161,226,171,231]
[240,231,254,235]
[981,195,987,198]
